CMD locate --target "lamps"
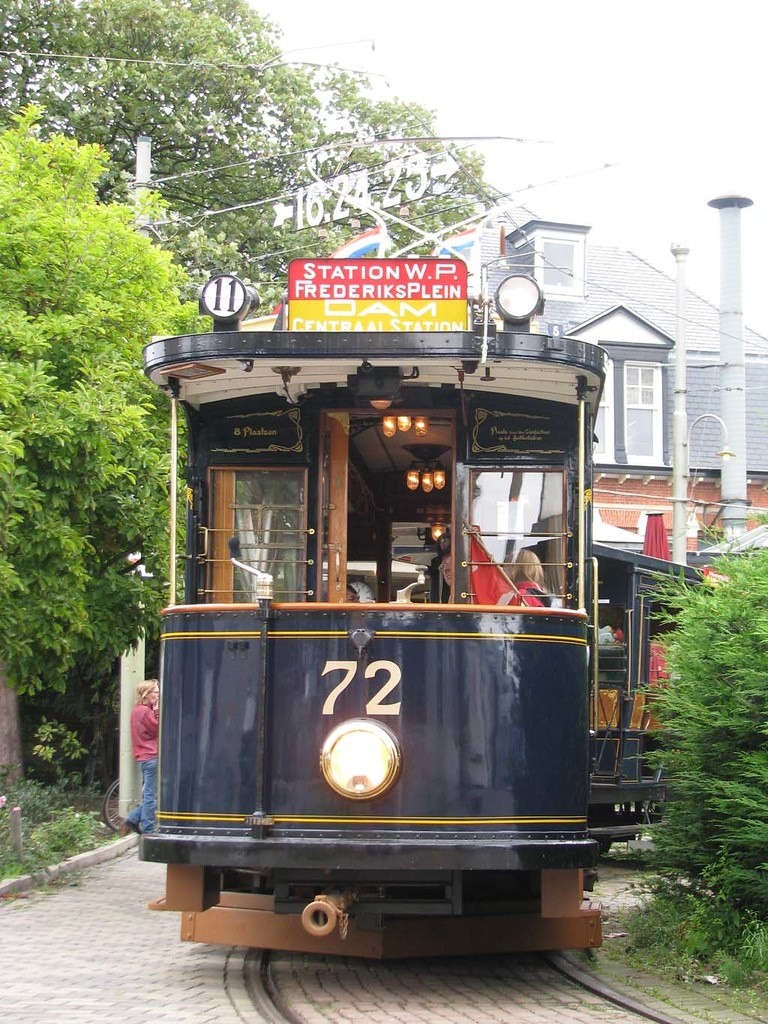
[414,416,429,438]
[422,471,434,493]
[407,469,420,491]
[431,523,446,541]
[434,469,446,489]
[398,416,412,432]
[383,416,397,438]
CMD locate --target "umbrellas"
[642,511,671,685]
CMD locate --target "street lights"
[687,411,737,492]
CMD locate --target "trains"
[136,257,729,958]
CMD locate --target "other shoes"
[124,819,142,835]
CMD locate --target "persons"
[124,679,160,834]
[438,552,451,604]
[346,584,360,603]
[502,549,558,608]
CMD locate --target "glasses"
[347,595,360,600]
[438,559,449,570]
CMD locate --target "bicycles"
[103,776,145,832]
